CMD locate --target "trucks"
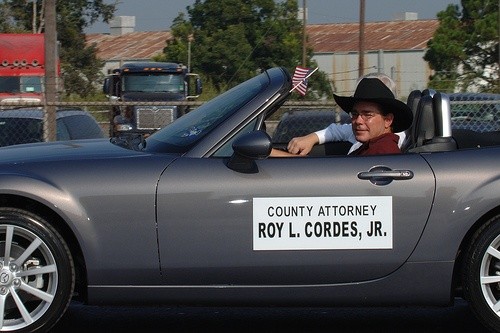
[104,60,203,150]
[0,29,59,112]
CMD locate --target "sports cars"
[0,66,500,333]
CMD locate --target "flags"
[292,67,312,94]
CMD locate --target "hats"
[333,78,414,133]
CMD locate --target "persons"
[288,73,408,155]
[268,77,414,156]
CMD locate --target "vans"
[0,96,105,147]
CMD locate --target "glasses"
[349,111,384,120]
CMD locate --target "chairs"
[398,88,457,153]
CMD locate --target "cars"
[274,93,500,149]
